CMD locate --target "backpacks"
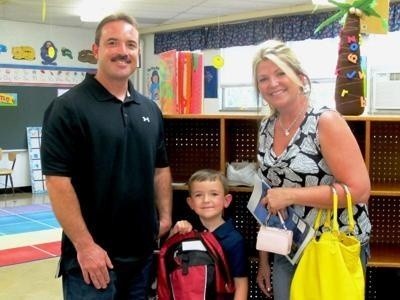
[155,228,237,300]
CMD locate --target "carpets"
[0,203,64,267]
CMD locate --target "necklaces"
[278,112,301,136]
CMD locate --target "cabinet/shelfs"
[161,114,400,300]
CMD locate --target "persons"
[41,12,172,300]
[252,39,371,300]
[40,41,58,64]
[165,169,248,300]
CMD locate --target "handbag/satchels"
[255,210,294,257]
[290,182,366,300]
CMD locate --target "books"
[246,176,316,267]
[145,48,204,115]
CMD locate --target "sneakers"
[225,161,259,187]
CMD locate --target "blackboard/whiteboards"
[0,83,79,151]
[276,211,289,232]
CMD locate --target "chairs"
[0,152,17,196]
